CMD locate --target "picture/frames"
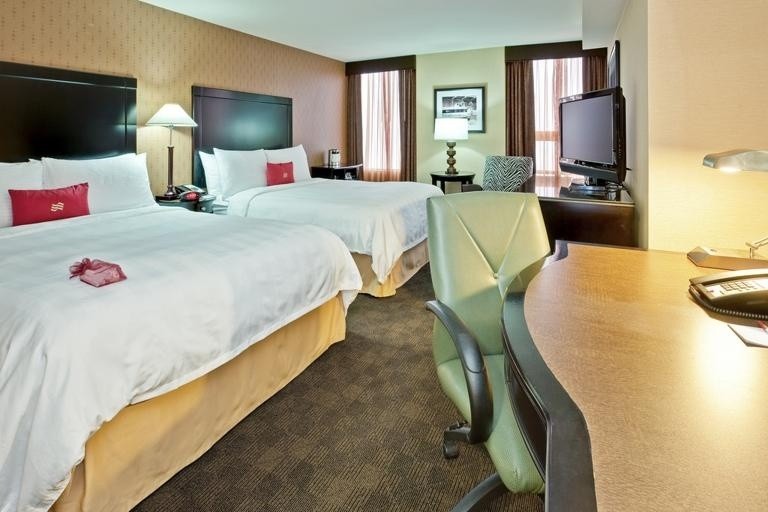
[434,86,486,133]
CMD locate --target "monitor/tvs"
[557,86,627,195]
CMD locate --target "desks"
[538,178,636,246]
[499,238,768,512]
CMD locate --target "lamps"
[685,148,768,271]
[433,117,470,175]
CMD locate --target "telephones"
[688,268,767,313]
[175,184,205,195]
